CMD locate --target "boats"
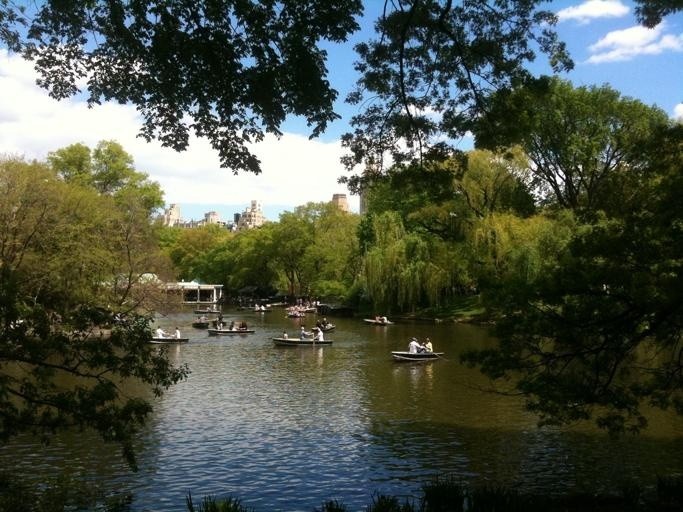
[273,337,334,346]
[319,322,336,332]
[241,301,288,312]
[390,351,445,361]
[207,327,256,335]
[212,318,226,326]
[364,319,394,325]
[194,309,220,313]
[192,319,209,327]
[148,336,189,344]
[285,303,317,317]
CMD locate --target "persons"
[407,336,433,353]
[375,315,387,323]
[282,317,330,341]
[193,313,247,333]
[156,326,165,337]
[173,327,180,338]
